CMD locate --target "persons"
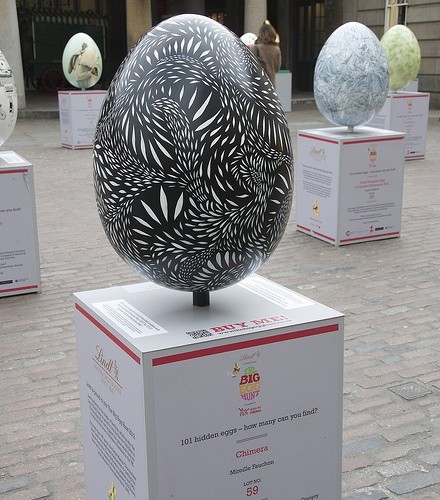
[251,20,283,91]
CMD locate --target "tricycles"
[32,61,66,92]
